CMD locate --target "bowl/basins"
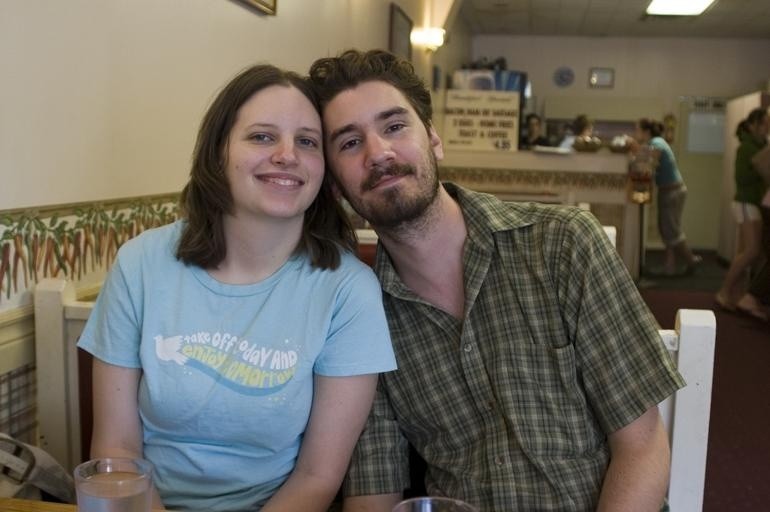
[610,145,631,153]
[571,142,602,152]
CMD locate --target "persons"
[523,113,552,145]
[75,62,400,512]
[559,113,594,150]
[301,45,688,512]
[714,105,770,311]
[737,143,770,322]
[626,116,701,273]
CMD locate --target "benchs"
[33,279,716,510]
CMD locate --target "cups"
[74,458,153,511]
[393,496,478,512]
